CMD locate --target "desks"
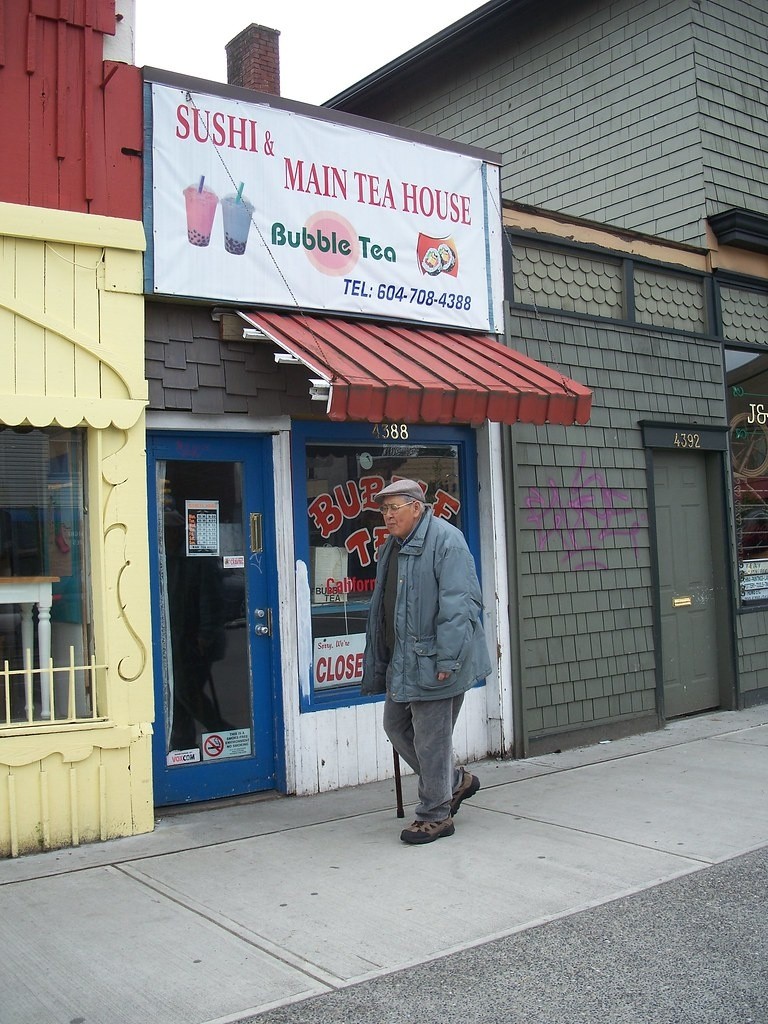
[0,576,61,720]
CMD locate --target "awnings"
[237,312,592,427]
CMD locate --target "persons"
[163,512,235,751]
[360,479,492,844]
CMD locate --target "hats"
[373,479,426,502]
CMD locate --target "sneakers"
[451,766,481,817]
[400,813,455,844]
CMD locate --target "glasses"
[379,501,414,515]
